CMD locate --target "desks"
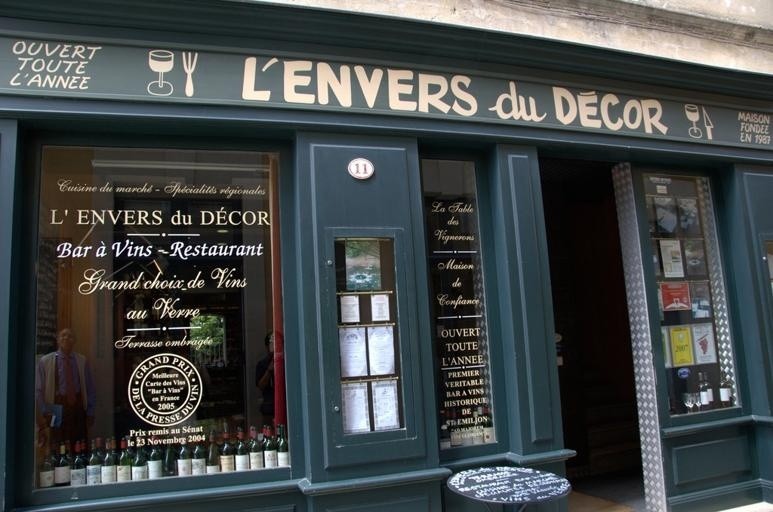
[447,464,571,511]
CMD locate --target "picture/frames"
[654,196,718,369]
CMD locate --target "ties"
[64,354,77,407]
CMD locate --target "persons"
[35,328,98,447]
[255,331,274,426]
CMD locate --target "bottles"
[446,406,495,447]
[717,371,735,410]
[39,419,289,488]
[698,371,715,412]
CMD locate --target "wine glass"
[680,391,696,414]
